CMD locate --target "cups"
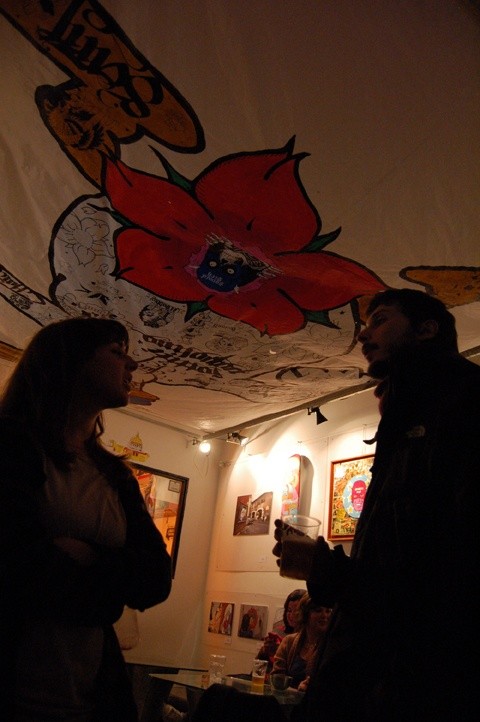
[279,514,321,581]
[251,659,268,687]
[207,653,226,686]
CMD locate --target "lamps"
[227,432,248,446]
[307,407,328,425]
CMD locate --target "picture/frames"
[328,453,375,541]
[126,460,190,579]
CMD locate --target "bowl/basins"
[272,675,292,690]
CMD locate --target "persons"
[162,589,332,722]
[273,288,480,722]
[0,317,173,722]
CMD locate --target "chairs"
[195,682,287,722]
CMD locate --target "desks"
[128,662,208,722]
[148,673,306,705]
